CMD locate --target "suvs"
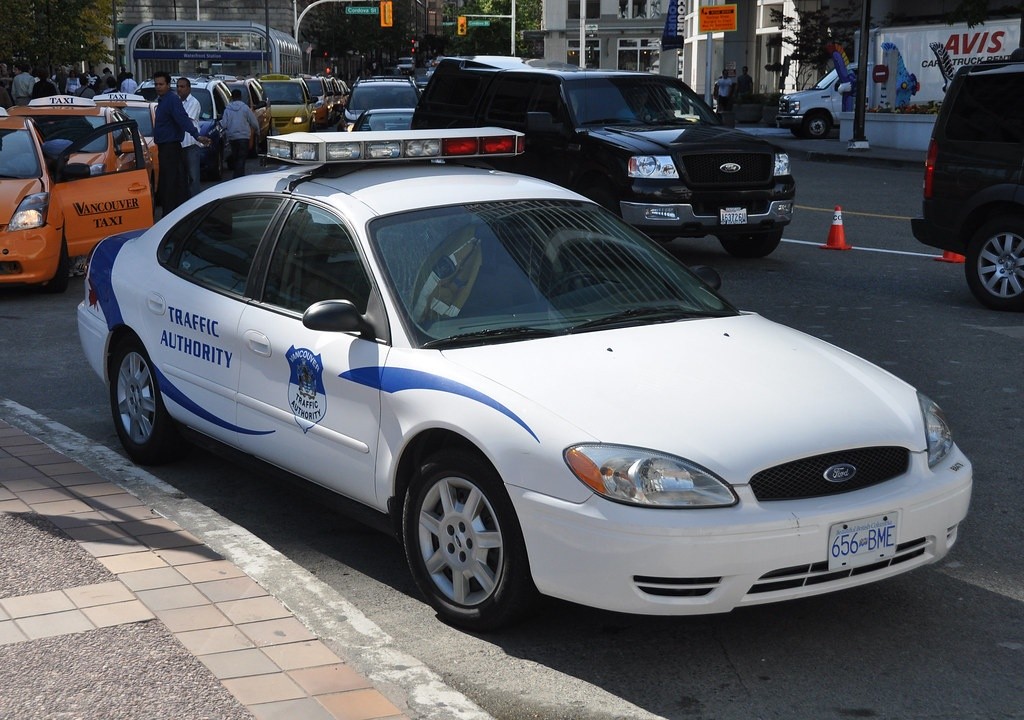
[332,75,422,132]
[134,75,233,181]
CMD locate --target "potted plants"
[717,100,736,128]
[734,96,765,123]
[764,92,782,124]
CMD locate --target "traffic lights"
[458,17,466,35]
[381,1,392,27]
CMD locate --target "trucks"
[776,18,1022,139]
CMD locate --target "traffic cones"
[933,249,965,262]
[821,206,852,250]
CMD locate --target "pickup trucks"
[410,55,796,257]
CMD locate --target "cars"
[81,101,160,192]
[78,156,973,631]
[257,77,319,134]
[398,57,415,75]
[6,105,155,212]
[416,56,444,88]
[0,116,153,292]
[305,77,351,127]
[911,61,1024,313]
[224,78,272,157]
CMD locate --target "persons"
[735,66,753,99]
[176,77,204,198]
[715,69,732,111]
[153,70,211,219]
[220,89,260,179]
[0,61,138,110]
[616,85,674,120]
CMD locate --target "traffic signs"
[346,7,379,14]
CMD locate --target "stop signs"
[872,65,889,84]
